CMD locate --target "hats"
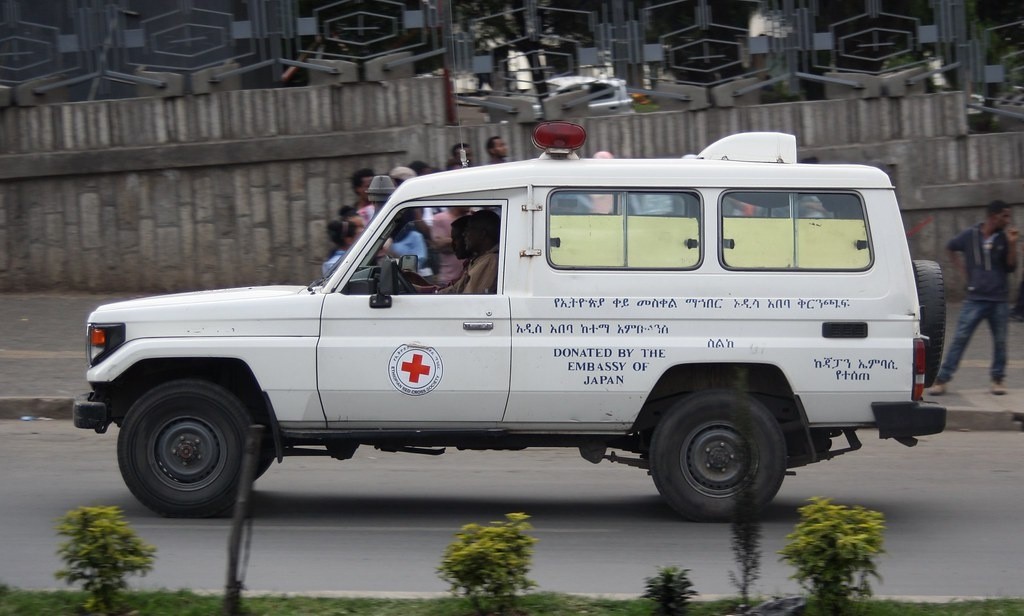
[390,166,417,181]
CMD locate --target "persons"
[436,209,501,295]
[411,214,472,295]
[321,135,889,278]
[928,199,1020,396]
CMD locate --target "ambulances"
[73,121,947,522]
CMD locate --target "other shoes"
[929,384,945,395]
[991,380,1006,394]
[1008,306,1024,322]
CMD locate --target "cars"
[521,76,635,119]
